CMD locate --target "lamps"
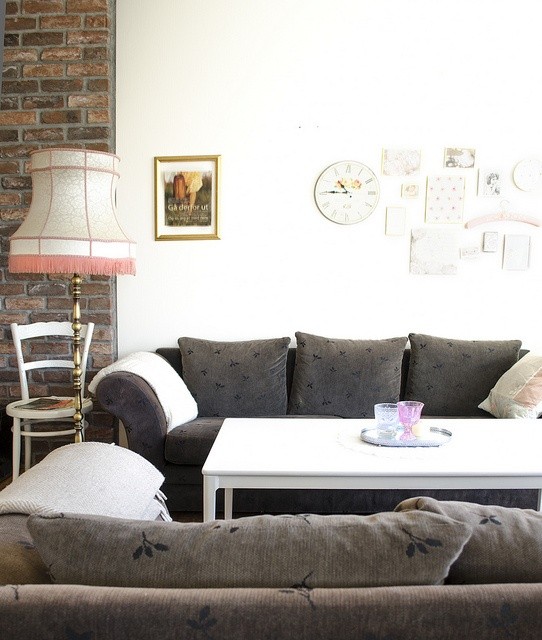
[8,148,137,441]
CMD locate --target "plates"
[358,427,453,448]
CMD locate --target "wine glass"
[396,400,424,443]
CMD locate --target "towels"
[88,352,198,433]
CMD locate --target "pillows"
[393,496,541,584]
[408,333,522,414]
[27,512,474,586]
[178,336,291,418]
[289,332,408,416]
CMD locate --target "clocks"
[314,160,380,224]
[155,154,222,241]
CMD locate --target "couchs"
[2,443,540,640]
[98,334,539,515]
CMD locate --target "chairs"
[6,320,95,480]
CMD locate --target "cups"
[373,403,401,439]
